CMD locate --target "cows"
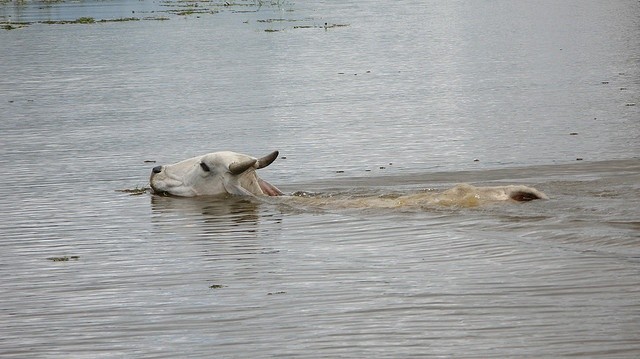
[149,150,551,214]
